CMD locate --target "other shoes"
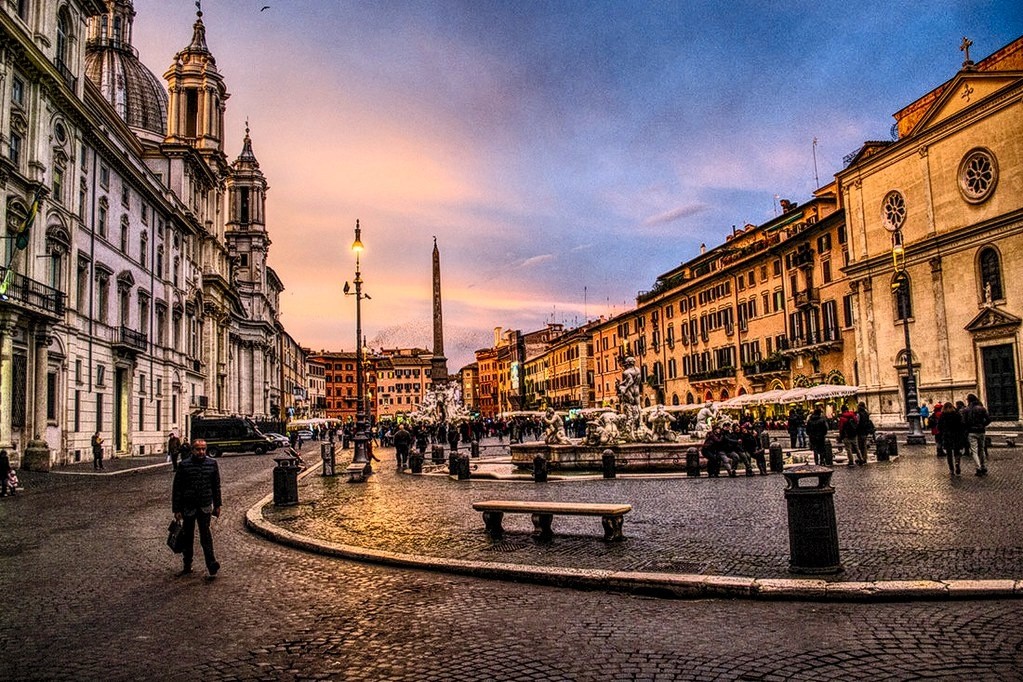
[731,469,736,476]
[761,471,768,475]
[746,474,751,476]
[208,562,220,575]
[937,453,946,456]
[955,463,961,476]
[708,475,714,477]
[730,474,736,477]
[858,459,864,465]
[714,474,720,478]
[751,472,755,476]
[975,473,982,477]
[178,568,192,574]
[847,461,853,466]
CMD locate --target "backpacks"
[928,412,938,429]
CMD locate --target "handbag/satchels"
[97,449,103,459]
[840,416,857,439]
[167,519,183,554]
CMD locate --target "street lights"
[344,219,382,477]
[888,228,927,446]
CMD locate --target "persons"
[647,403,676,441]
[615,357,642,411]
[697,399,719,431]
[541,407,572,443]
[91,432,105,470]
[920,393,990,478]
[0,450,12,497]
[557,401,875,479]
[166,438,222,577]
[288,416,547,468]
[167,433,190,473]
[595,407,621,439]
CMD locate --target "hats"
[857,402,866,409]
[169,433,174,436]
[11,470,16,474]
[934,403,943,408]
[814,409,823,414]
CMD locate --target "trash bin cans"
[343,435,349,449]
[273,466,301,506]
[783,470,843,575]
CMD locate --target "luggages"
[824,441,833,465]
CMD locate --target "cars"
[263,432,290,448]
[298,430,313,440]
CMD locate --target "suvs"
[191,414,277,456]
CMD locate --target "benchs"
[973,434,1019,447]
[473,499,632,542]
[347,462,369,481]
